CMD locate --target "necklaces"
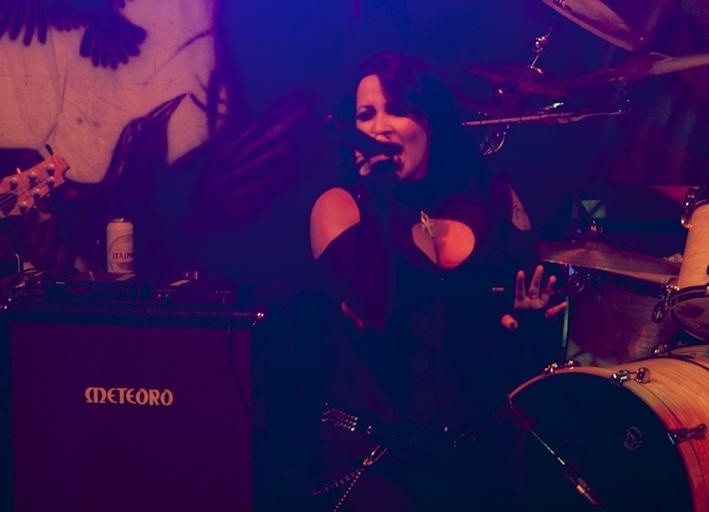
[418,209,435,240]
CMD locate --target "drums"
[510,353,709,511]
[663,193,709,340]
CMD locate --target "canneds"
[107,218,136,276]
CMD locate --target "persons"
[308,51,569,509]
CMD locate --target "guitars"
[1,155,69,217]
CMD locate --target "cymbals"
[537,236,679,286]
[467,96,620,131]
[474,66,577,102]
[550,0,639,50]
[571,53,706,83]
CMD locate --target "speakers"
[4,299,259,510]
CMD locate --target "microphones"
[337,129,402,157]
[535,36,554,52]
